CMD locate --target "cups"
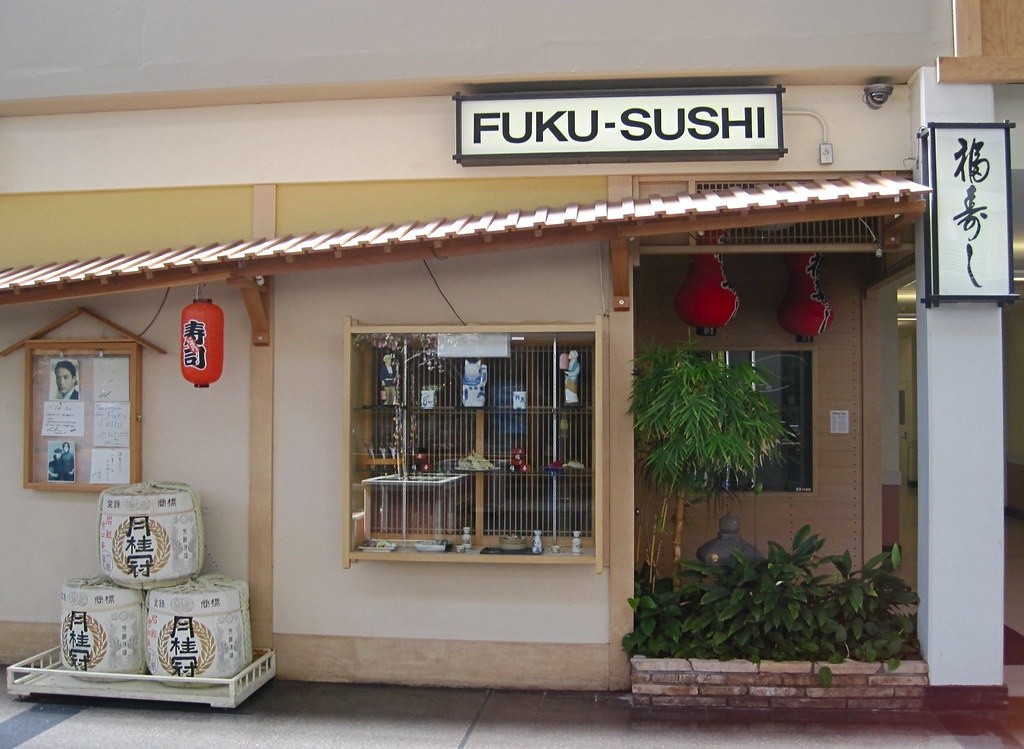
[551,546,561,553]
[420,390,438,410]
[456,546,465,553]
[512,391,528,410]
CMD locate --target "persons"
[49,442,74,480]
[380,354,400,405]
[55,361,78,399]
[564,350,580,406]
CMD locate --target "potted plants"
[622,326,928,716]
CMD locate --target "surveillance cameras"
[863,83,894,106]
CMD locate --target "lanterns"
[675,230,740,336]
[181,298,224,388]
[777,253,833,343]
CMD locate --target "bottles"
[532,530,542,554]
[571,531,582,553]
[462,527,472,549]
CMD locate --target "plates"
[454,467,501,471]
[358,546,396,553]
[415,542,447,552]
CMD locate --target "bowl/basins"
[499,536,527,550]
